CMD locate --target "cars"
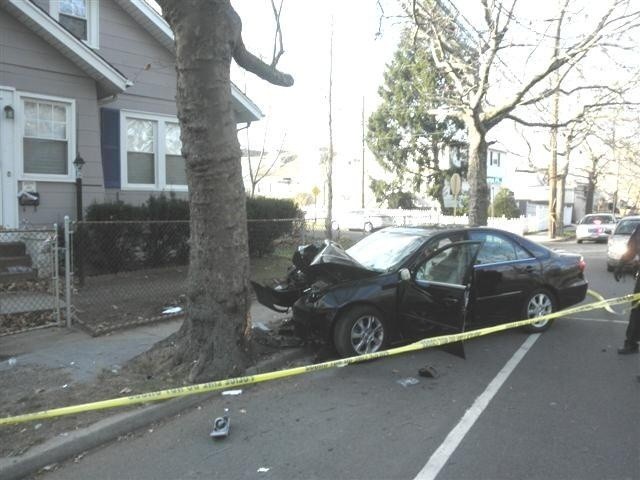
[606,217,640,272]
[248,223,589,361]
[574,214,617,244]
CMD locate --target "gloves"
[614,257,627,281]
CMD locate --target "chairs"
[429,238,468,285]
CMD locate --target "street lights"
[72,151,87,222]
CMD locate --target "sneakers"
[618,340,639,354]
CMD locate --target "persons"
[612,225,640,355]
